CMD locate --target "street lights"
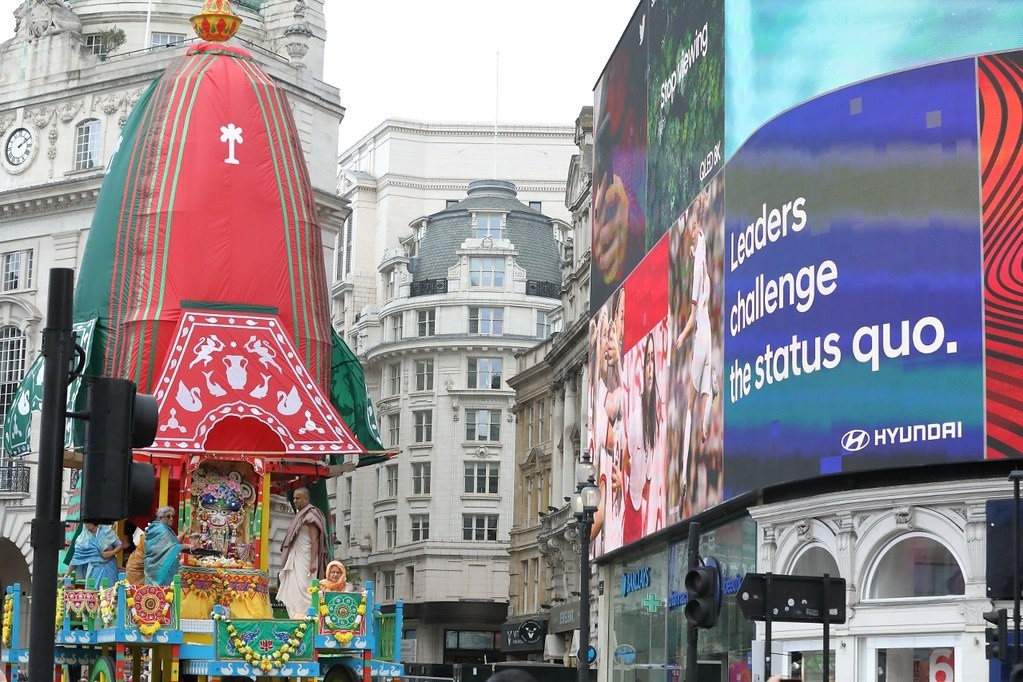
[570,450,601,681]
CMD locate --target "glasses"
[160,514,174,519]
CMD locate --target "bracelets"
[64,574,68,578]
[112,550,115,555]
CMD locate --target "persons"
[587,196,720,554]
[125,506,194,587]
[317,560,353,592]
[274,486,328,620]
[60,523,122,630]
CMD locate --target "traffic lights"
[81,378,158,523]
[982,610,1007,660]
[684,565,716,629]
